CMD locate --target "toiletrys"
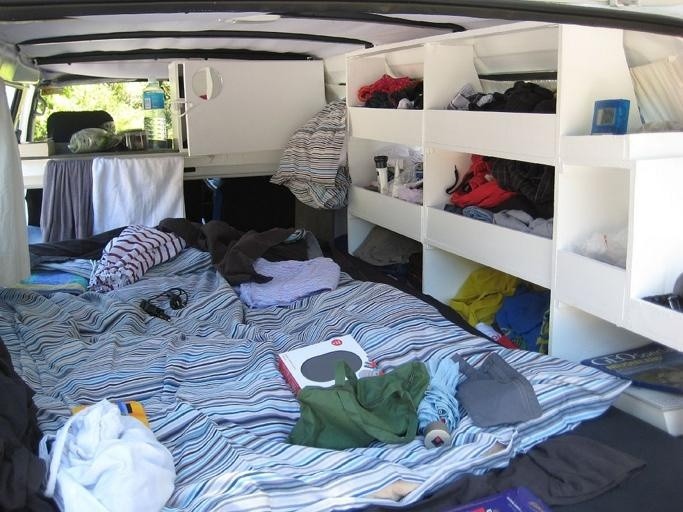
[393,159,400,199]
[374,154,389,194]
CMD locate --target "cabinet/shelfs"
[343,19,683,439]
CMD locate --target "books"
[277,333,380,396]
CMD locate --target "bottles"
[141,73,169,151]
[372,155,390,193]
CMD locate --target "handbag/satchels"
[39,397,177,512]
[290,361,430,448]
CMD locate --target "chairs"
[45,110,114,143]
